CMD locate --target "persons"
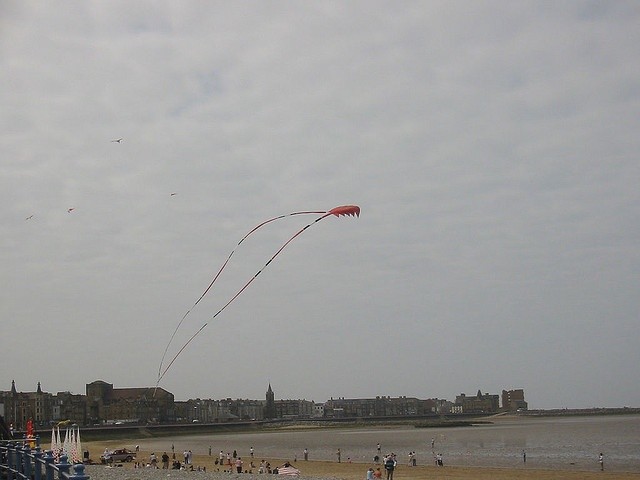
[150,453,156,468]
[283,461,292,468]
[161,452,170,470]
[303,448,309,461]
[384,453,397,480]
[431,438,435,448]
[406,452,412,468]
[219,450,224,465]
[336,448,340,463]
[226,452,231,464]
[215,457,219,465]
[172,460,186,471]
[435,453,439,464]
[521,450,526,462]
[104,448,112,467]
[431,452,436,465]
[170,442,175,452]
[179,466,196,471]
[141,459,145,468]
[234,457,244,473]
[598,452,604,471]
[375,467,382,480]
[249,446,254,458]
[376,442,382,451]
[135,461,141,470]
[412,451,417,466]
[183,450,187,463]
[366,467,373,480]
[135,445,139,451]
[273,467,280,474]
[188,450,192,463]
[209,446,212,455]
[249,470,252,473]
[248,462,257,468]
[260,460,272,473]
[439,453,443,467]
[232,449,238,462]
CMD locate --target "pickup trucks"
[100,449,137,463]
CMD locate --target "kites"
[68,208,76,215]
[329,205,360,218]
[24,213,36,221]
[170,193,175,197]
